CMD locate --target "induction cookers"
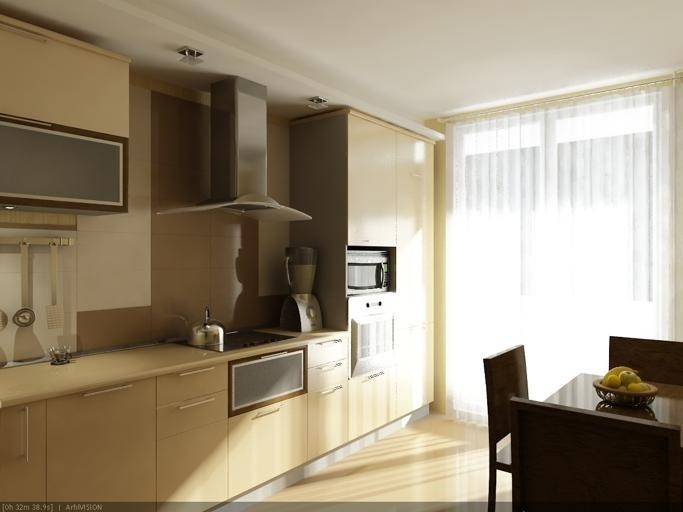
[169,327,293,353]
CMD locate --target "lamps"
[305,96,328,110]
[175,46,204,67]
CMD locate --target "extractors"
[157,74,312,225]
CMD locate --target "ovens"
[347,298,398,376]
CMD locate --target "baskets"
[593,378,659,407]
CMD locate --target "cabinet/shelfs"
[397,132,435,419]
[228,393,308,500]
[308,331,348,461]
[348,364,397,442]
[0,393,46,511]
[157,356,228,511]
[290,114,397,246]
[47,370,157,512]
[0,14,131,139]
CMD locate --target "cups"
[47,343,69,363]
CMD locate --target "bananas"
[603,366,639,382]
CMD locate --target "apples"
[608,370,652,392]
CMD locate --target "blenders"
[279,243,323,332]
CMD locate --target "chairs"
[510,397,681,512]
[483,344,529,512]
[609,335,683,386]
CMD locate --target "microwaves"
[344,248,389,296]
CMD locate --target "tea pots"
[185,305,224,347]
